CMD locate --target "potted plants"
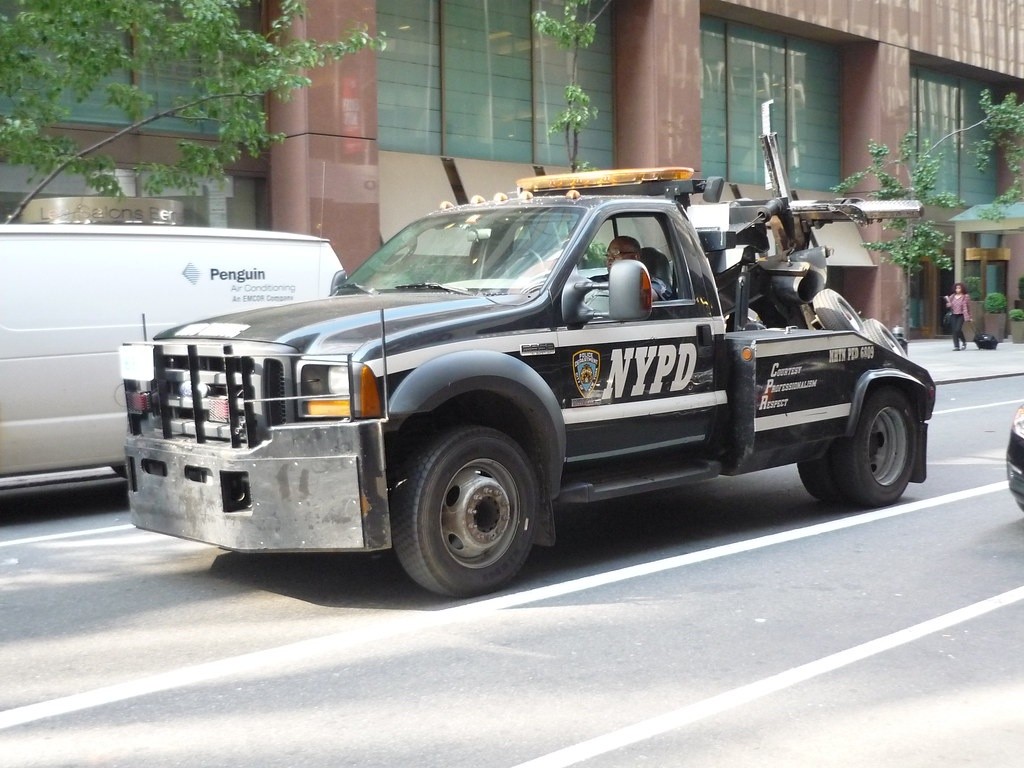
[1009,310,1024,344]
[961,277,984,342]
[984,293,1007,341]
[1014,277,1024,310]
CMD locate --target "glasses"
[601,248,635,257]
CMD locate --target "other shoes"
[952,346,959,351]
[961,343,966,351]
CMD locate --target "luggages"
[968,319,998,350]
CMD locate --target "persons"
[587,235,676,303]
[944,283,974,351]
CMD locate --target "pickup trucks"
[118,164,936,597]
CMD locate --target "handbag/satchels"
[942,311,952,326]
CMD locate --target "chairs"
[637,247,672,300]
